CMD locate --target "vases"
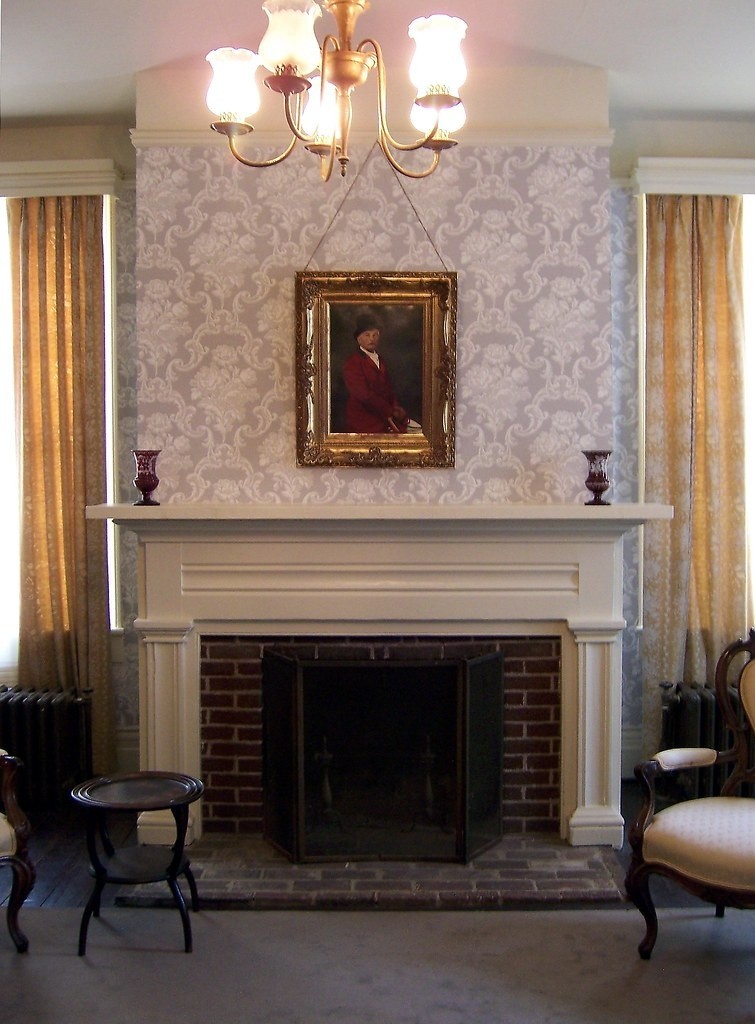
[131,449,162,506]
[580,448,614,505]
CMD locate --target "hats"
[353,315,381,338]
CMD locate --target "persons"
[341,314,410,434]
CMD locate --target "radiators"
[0,683,95,836]
[657,680,739,802]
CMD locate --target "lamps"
[205,0,468,183]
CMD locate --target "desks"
[71,771,203,957]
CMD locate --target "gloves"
[393,406,405,420]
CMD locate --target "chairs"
[622,626,755,962]
[0,749,36,953]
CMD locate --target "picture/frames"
[294,269,456,470]
